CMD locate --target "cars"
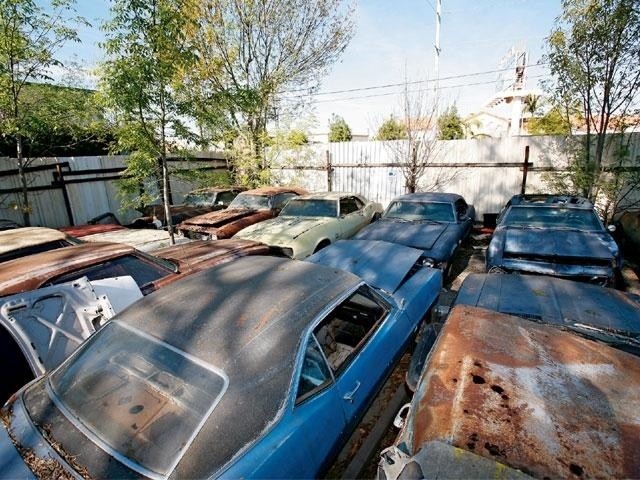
[372,267,639,480]
[485,191,622,287]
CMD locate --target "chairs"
[300,347,330,387]
